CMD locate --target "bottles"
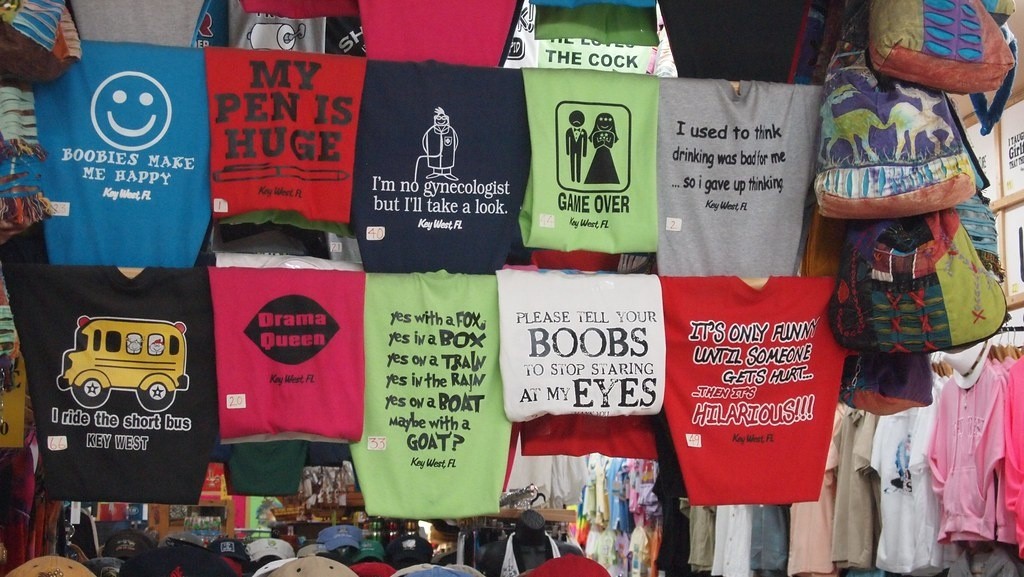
[372,520,417,544]
[362,524,370,541]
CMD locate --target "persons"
[475,509,584,577]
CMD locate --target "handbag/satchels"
[0,0,82,241]
[813,0,1015,416]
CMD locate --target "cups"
[184,516,223,547]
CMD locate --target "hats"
[3,525,611,577]
